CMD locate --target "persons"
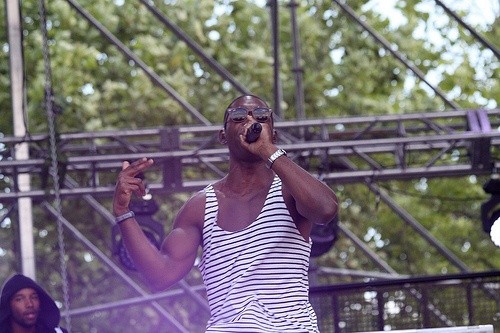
[113,92,339,333]
[0,275,69,333]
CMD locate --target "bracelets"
[115,211,135,224]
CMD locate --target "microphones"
[245,123,262,143]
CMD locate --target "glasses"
[225,107,273,127]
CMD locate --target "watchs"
[264,147,287,171]
